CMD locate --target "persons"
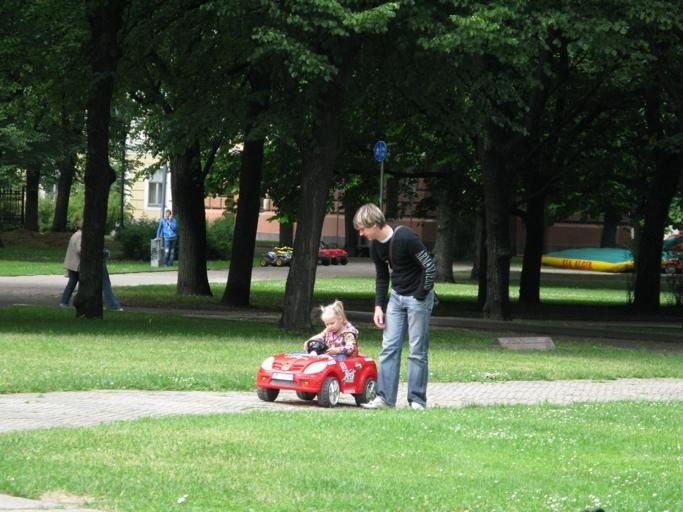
[155,210,176,266]
[352,204,437,409]
[301,301,359,358]
[100,244,123,312]
[59,228,82,303]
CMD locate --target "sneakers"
[409,401,426,414]
[60,303,125,314]
[359,396,395,410]
